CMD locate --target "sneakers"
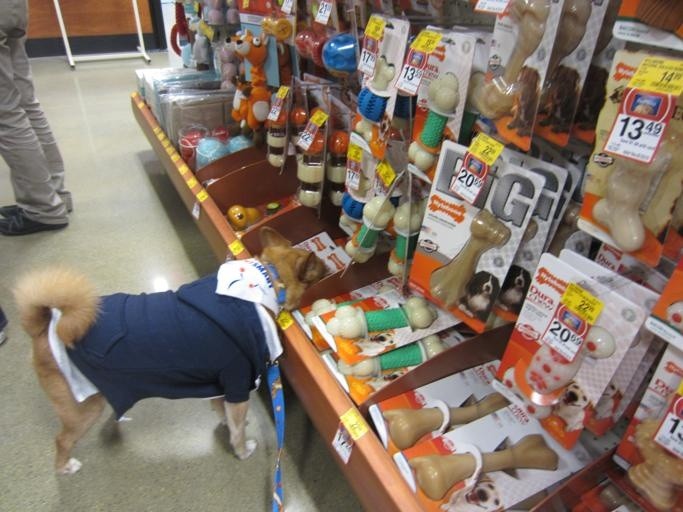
[0,204,74,235]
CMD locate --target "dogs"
[10,225,330,476]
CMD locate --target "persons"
[0,0,74,237]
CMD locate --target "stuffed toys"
[226,28,251,124]
[229,26,272,132]
